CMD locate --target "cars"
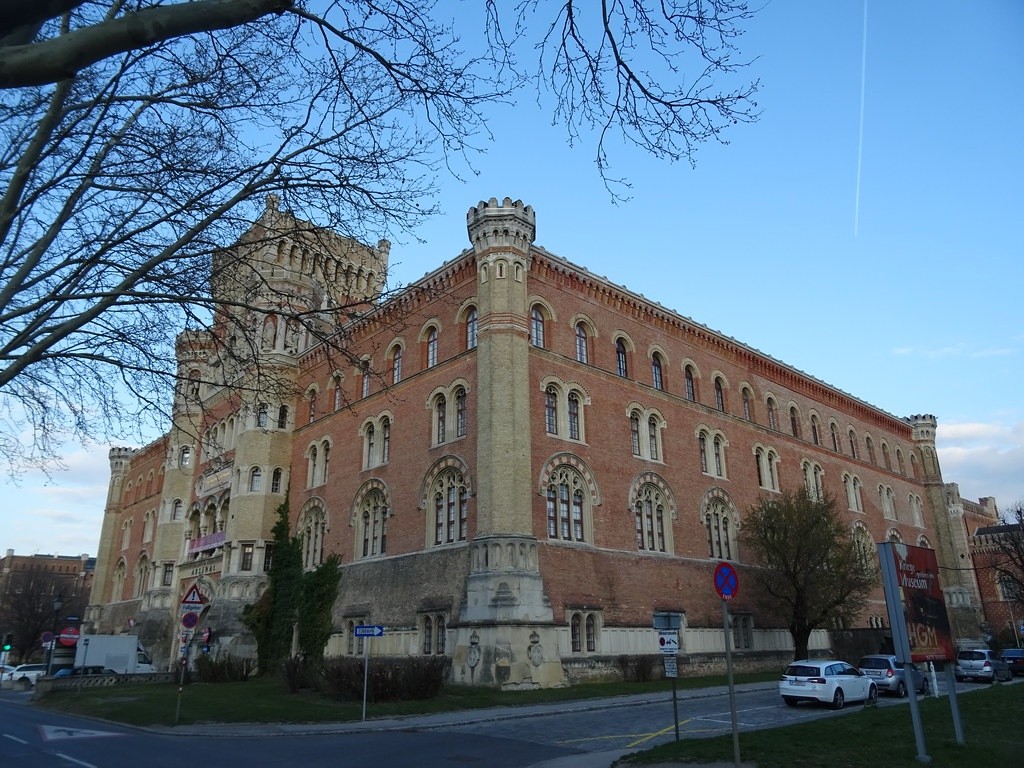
[998,648,1024,675]
[53,669,72,679]
[0,665,16,682]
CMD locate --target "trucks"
[73,634,161,675]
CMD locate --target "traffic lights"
[3,633,14,651]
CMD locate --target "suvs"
[7,664,48,687]
[779,660,878,711]
[955,648,1012,684]
[857,654,929,698]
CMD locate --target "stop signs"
[59,626,79,646]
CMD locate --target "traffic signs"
[355,625,383,636]
